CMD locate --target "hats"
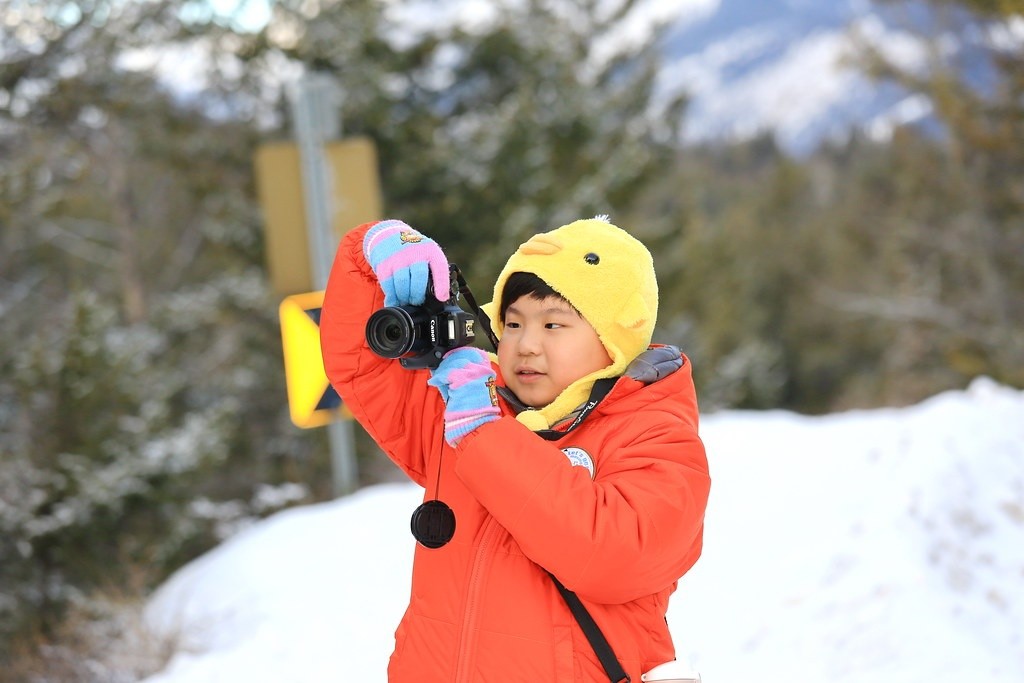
[479,217,659,433]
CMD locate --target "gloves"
[362,220,451,309]
[427,346,501,449]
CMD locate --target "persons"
[315,213,712,683]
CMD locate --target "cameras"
[365,296,473,369]
